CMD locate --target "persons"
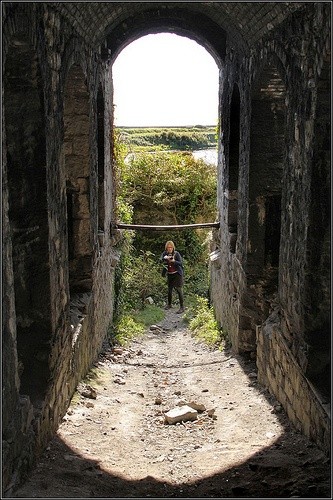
[159,240,185,314]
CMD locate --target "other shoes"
[177,307,186,313]
[164,303,172,309]
